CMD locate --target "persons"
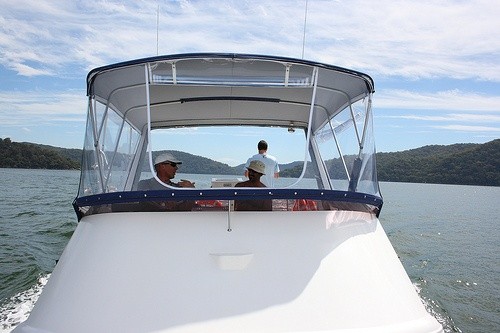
[138,152,196,208]
[233,160,273,211]
[244,140,280,188]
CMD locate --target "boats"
[8,0,444,333]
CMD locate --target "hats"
[154,153,182,165]
[246,160,266,175]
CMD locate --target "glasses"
[161,161,177,167]
